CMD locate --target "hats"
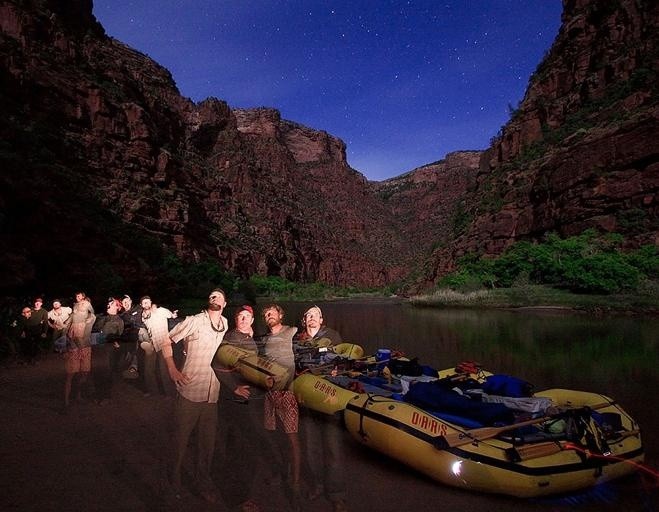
[108,294,131,312]
[302,305,323,319]
[234,304,254,317]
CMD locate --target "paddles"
[431,400,626,451]
[505,429,646,462]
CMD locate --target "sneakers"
[91,397,112,408]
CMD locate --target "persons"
[9,286,178,406]
[161,288,346,511]
